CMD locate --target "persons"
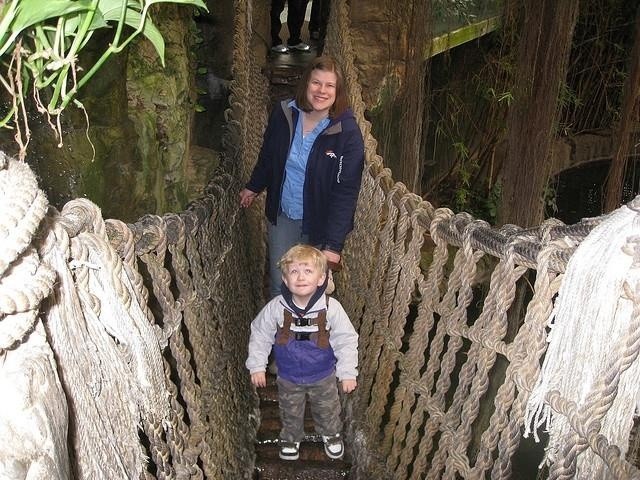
[239,56,365,298]
[245,244,361,461]
[270,0,311,53]
[308,0,327,41]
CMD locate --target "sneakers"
[272,44,289,52]
[322,433,344,459]
[288,42,311,50]
[279,441,300,460]
